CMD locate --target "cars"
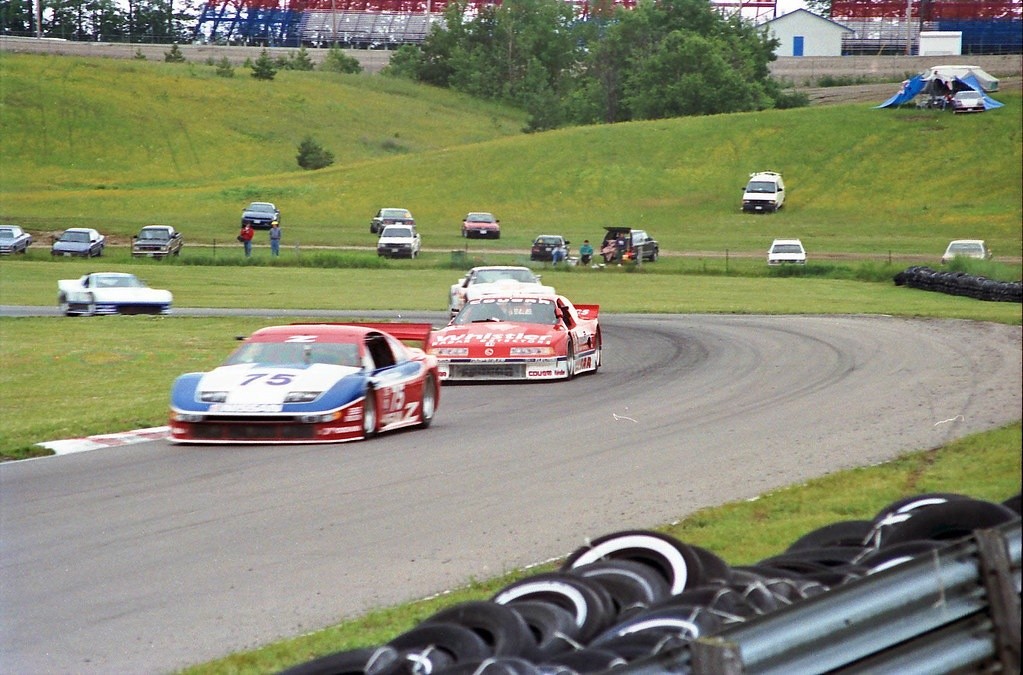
[530,235,571,261]
[941,239,993,265]
[55,271,173,317]
[51,228,107,259]
[241,202,281,230]
[766,239,807,266]
[460,212,501,239]
[0,225,33,256]
[370,208,416,234]
[376,222,421,259]
[449,266,555,320]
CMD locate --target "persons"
[580,240,594,265]
[270,222,281,256]
[551,242,567,264]
[615,231,627,267]
[241,222,255,258]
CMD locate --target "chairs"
[114,278,131,286]
[576,251,594,267]
[160,231,168,237]
[471,216,477,220]
[554,240,560,244]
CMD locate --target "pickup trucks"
[132,224,183,260]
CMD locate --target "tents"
[872,68,1004,111]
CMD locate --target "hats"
[271,221,279,225]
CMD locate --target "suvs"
[599,226,660,262]
[741,172,785,213]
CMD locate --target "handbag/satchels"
[237,236,244,242]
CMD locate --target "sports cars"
[423,292,603,381]
[166,320,440,445]
